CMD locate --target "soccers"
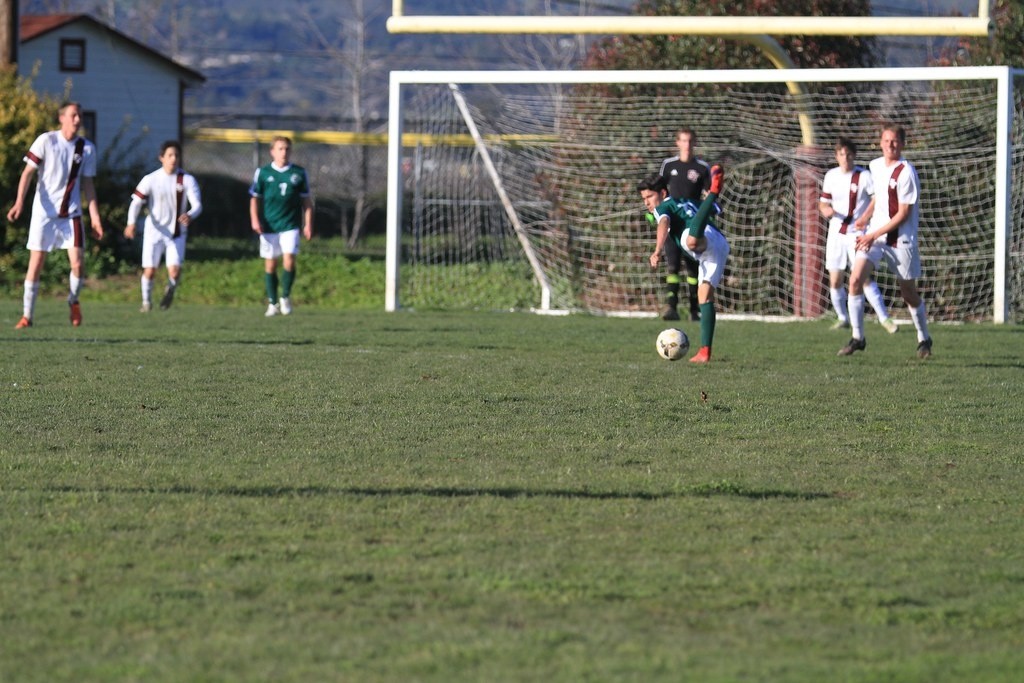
[657,327,690,362]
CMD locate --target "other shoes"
[663,307,680,320]
[831,319,848,329]
[883,317,897,333]
[139,304,152,312]
[691,313,701,321]
[160,292,173,311]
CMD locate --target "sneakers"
[917,336,932,358]
[836,337,866,357]
[279,297,291,315]
[706,163,725,194]
[68,300,82,327]
[689,348,710,362]
[265,303,280,317]
[15,316,32,329]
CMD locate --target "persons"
[819,139,898,335]
[636,164,730,364]
[646,128,712,322]
[123,140,202,312]
[838,123,933,358]
[7,102,103,329]
[248,136,313,317]
